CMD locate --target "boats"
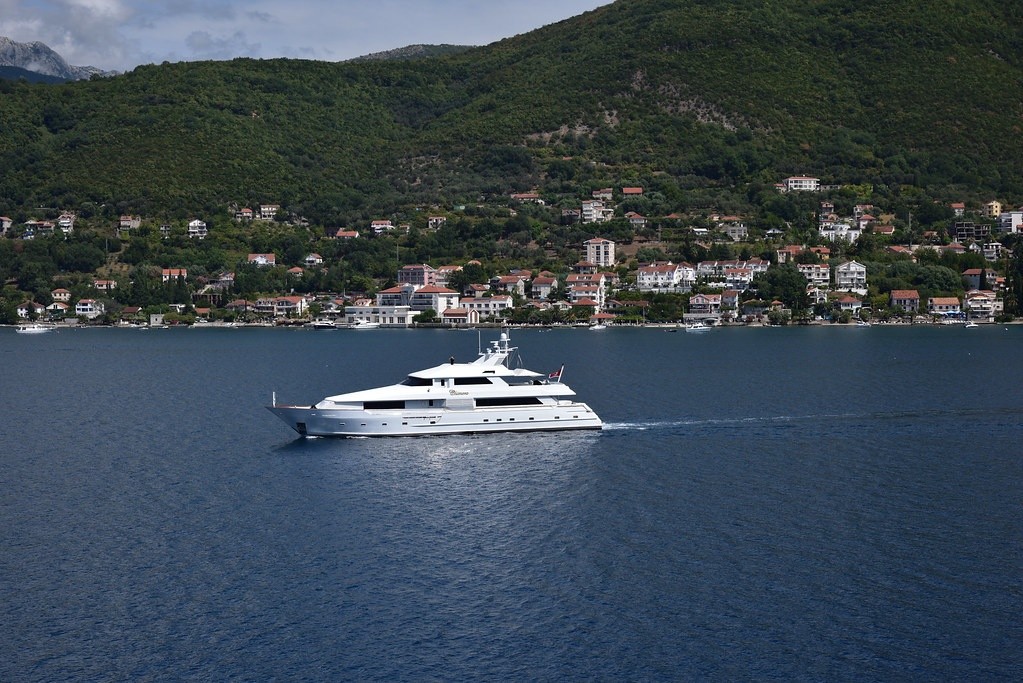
[588,323,607,331]
[262,317,605,440]
[685,321,712,332]
[348,314,381,329]
[15,323,56,334]
[963,321,979,328]
[313,315,338,330]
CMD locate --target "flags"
[549,368,561,378]
